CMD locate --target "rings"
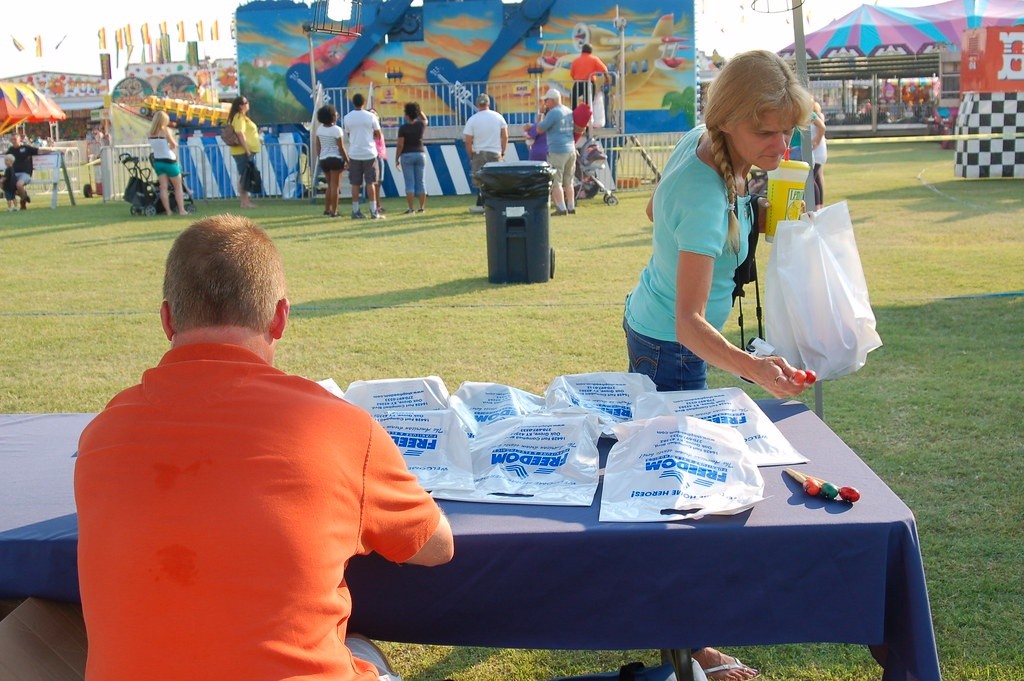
[775,376,782,386]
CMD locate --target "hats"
[540,89,561,101]
[476,93,490,106]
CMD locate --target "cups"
[765,159,811,243]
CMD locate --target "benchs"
[0,151,77,209]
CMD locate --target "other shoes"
[351,210,365,219]
[370,207,385,218]
[551,206,566,215]
[404,209,415,215]
[418,209,424,214]
[321,210,344,217]
[568,209,575,214]
[240,201,258,208]
[469,206,485,213]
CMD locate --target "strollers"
[118,152,196,218]
[577,133,620,207]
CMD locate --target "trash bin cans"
[481,161,555,285]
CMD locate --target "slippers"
[688,646,760,681]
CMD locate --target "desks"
[0,396,941,681]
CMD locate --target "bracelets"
[502,153,504,156]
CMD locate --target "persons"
[73,215,454,681]
[228,97,260,207]
[811,103,827,209]
[148,111,191,215]
[524,109,547,161]
[344,94,387,219]
[622,50,810,681]
[537,89,576,216]
[463,94,508,212]
[315,106,350,217]
[0,134,66,211]
[396,103,427,213]
[570,44,610,111]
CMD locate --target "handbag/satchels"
[221,111,246,146]
[313,372,810,523]
[762,200,883,382]
[240,158,261,193]
